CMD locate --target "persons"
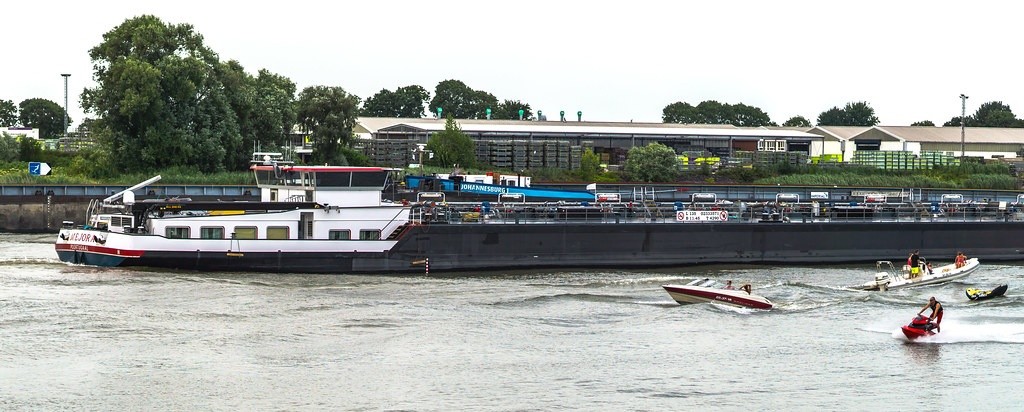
[739,284,751,295]
[955,253,967,269]
[917,297,943,332]
[722,281,735,290]
[908,249,934,278]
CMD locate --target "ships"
[55,140,1024,274]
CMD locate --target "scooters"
[901,313,938,341]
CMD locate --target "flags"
[586,183,595,190]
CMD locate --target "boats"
[863,258,980,293]
[966,283,1008,301]
[662,279,773,310]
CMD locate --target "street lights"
[959,93,969,155]
[62,73,70,148]
